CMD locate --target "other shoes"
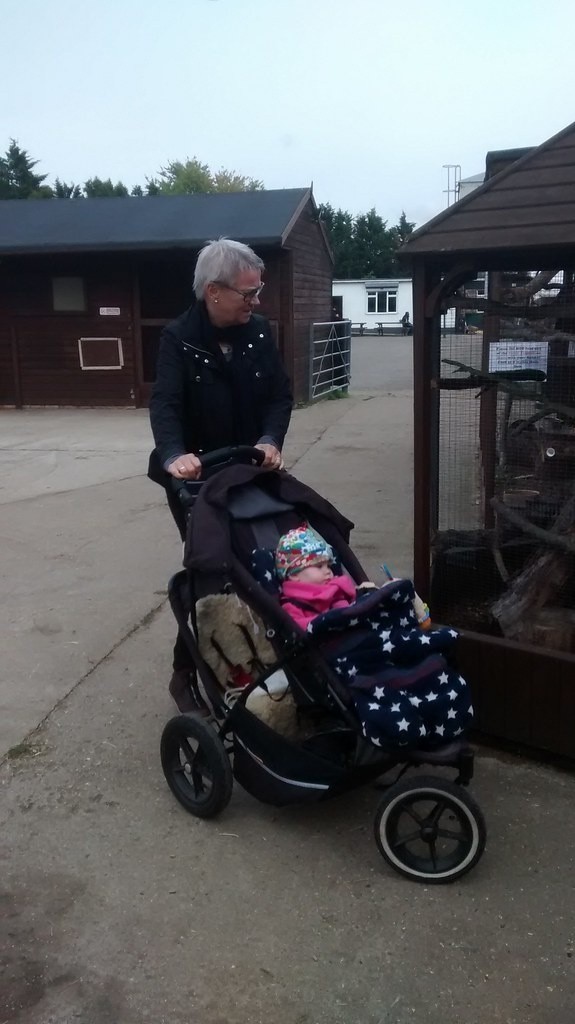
[168,670,213,726]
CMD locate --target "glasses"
[216,280,264,302]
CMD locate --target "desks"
[375,322,409,336]
[350,323,367,337]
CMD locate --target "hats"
[273,527,336,578]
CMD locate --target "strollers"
[159,447,490,883]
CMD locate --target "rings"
[178,466,185,471]
[276,457,281,460]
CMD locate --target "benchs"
[350,328,367,332]
[374,326,412,330]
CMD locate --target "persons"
[402,312,414,336]
[147,233,295,717]
[275,521,362,634]
